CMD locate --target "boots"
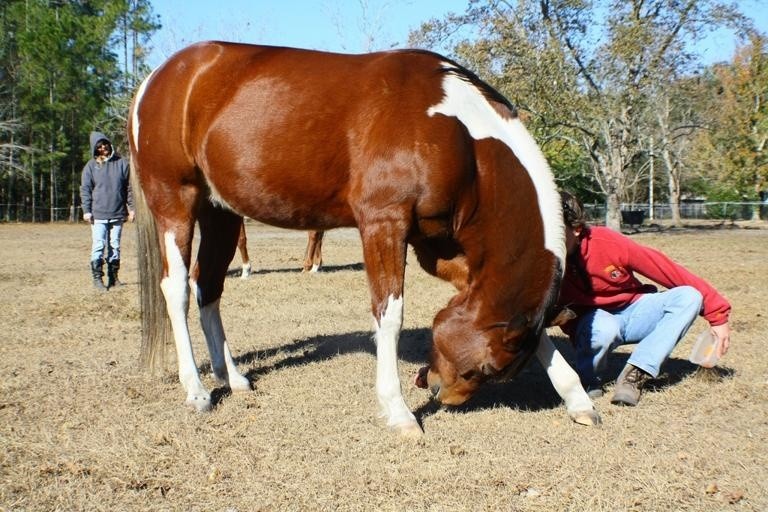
[105,258,120,290]
[91,258,107,291]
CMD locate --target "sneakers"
[610,364,646,405]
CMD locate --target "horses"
[120,38,600,448]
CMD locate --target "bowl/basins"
[688,330,720,369]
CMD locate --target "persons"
[79,131,135,291]
[557,189,732,409]
